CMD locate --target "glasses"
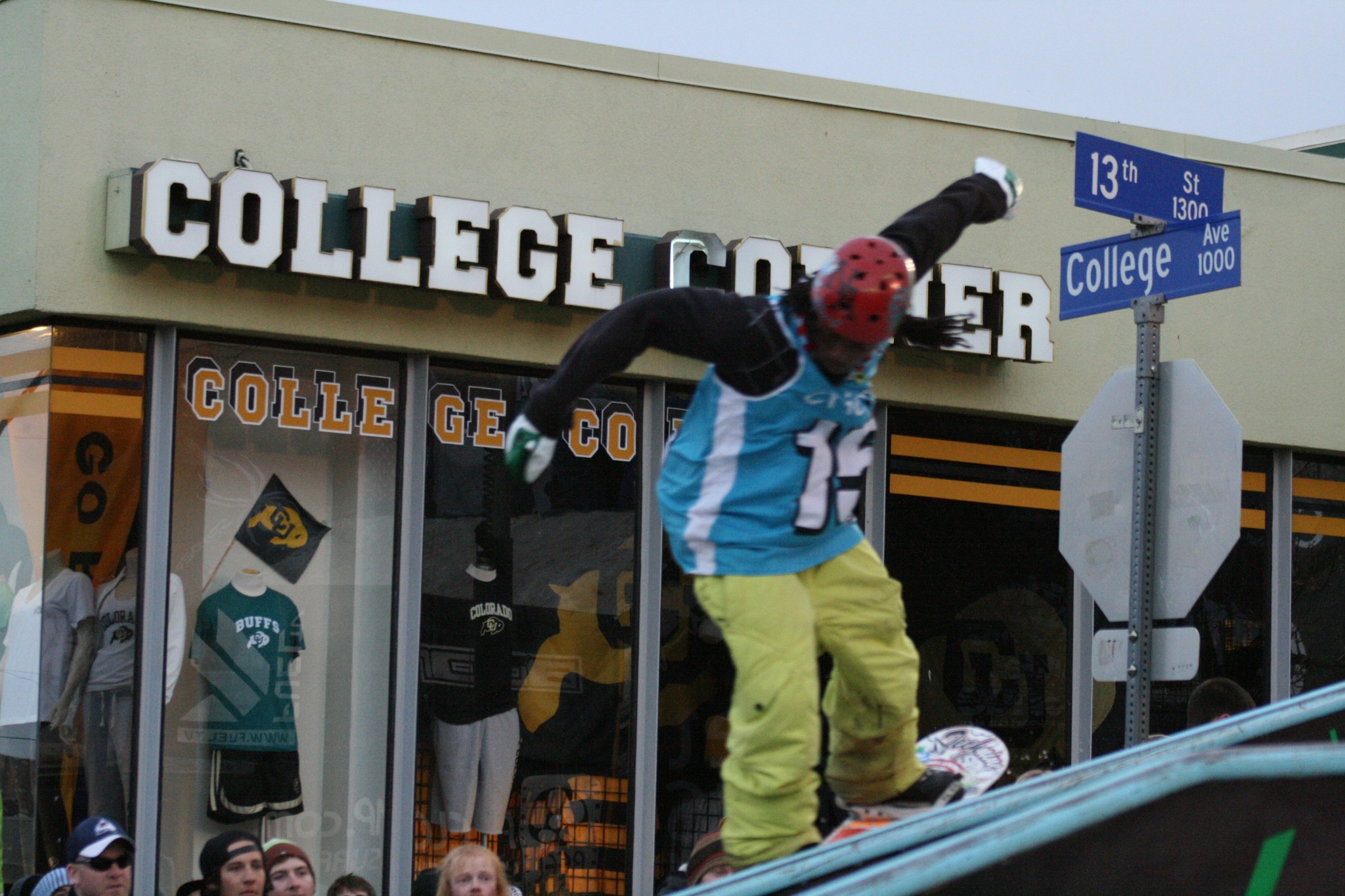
[72,857,135,871]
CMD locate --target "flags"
[235,474,330,583]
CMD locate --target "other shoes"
[833,765,964,818]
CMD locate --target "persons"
[0,550,97,867]
[503,156,1022,868]
[435,566,522,848]
[191,568,305,840]
[58,549,187,836]
[1185,679,1257,731]
[3,816,533,896]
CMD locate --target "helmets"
[809,231,917,345]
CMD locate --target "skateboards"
[819,722,1012,848]
[1186,676,1257,730]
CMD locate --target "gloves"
[973,156,1024,207]
[503,412,560,483]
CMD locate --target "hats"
[685,831,730,887]
[31,866,72,896]
[66,814,136,858]
[8,872,44,896]
[261,839,316,878]
[200,830,266,881]
